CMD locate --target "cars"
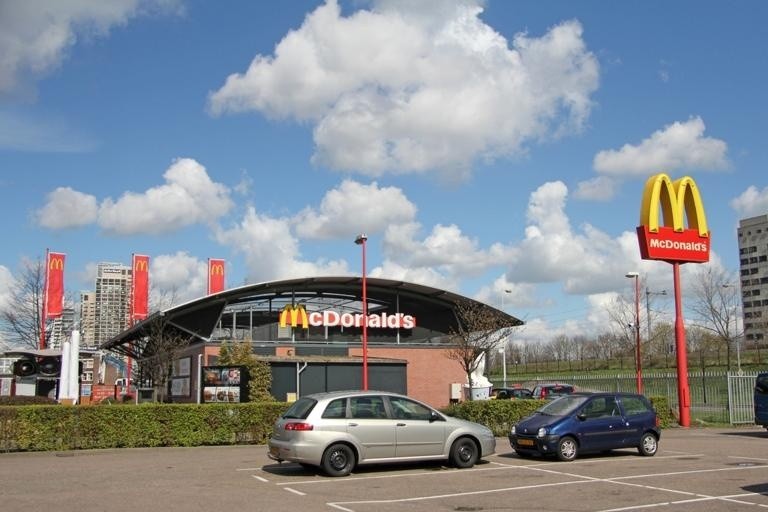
[492,388,532,399]
[266,389,495,477]
[507,392,661,462]
[529,384,578,400]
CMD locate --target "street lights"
[354,233,368,392]
[625,271,642,395]
[500,288,511,388]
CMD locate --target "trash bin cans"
[138,387,154,403]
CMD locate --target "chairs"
[356,398,385,417]
[584,401,620,415]
